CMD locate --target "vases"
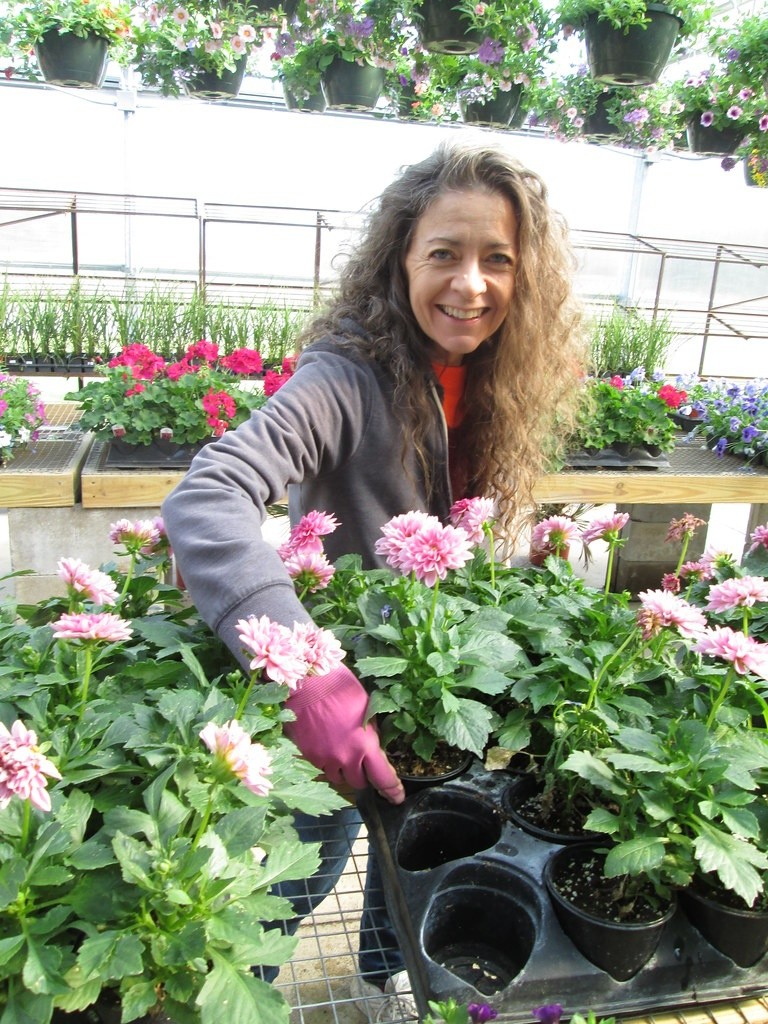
[278,72,326,114]
[685,109,747,157]
[459,83,522,128]
[386,746,474,797]
[509,102,528,129]
[612,441,632,457]
[219,0,298,28]
[643,441,662,457]
[35,24,113,90]
[668,409,704,432]
[544,843,675,982]
[684,887,767,968]
[397,81,445,120]
[320,53,387,111]
[583,3,685,86]
[743,157,767,187]
[183,53,248,99]
[503,775,610,844]
[413,1,485,55]
[580,444,600,457]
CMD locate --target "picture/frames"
[580,92,620,139]
[114,439,138,455]
[154,438,182,456]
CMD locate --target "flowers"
[544,364,768,473]
[64,342,301,447]
[1,498,768,1024]
[0,0,768,188]
[0,371,49,463]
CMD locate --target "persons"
[160,147,584,1024]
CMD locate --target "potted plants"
[586,300,698,380]
[0,271,306,377]
